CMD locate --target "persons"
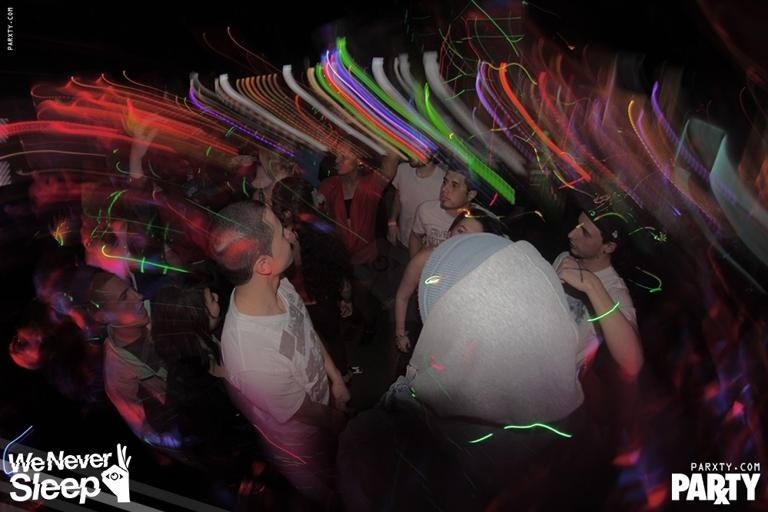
[0,122,643,512]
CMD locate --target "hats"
[418,233,514,325]
